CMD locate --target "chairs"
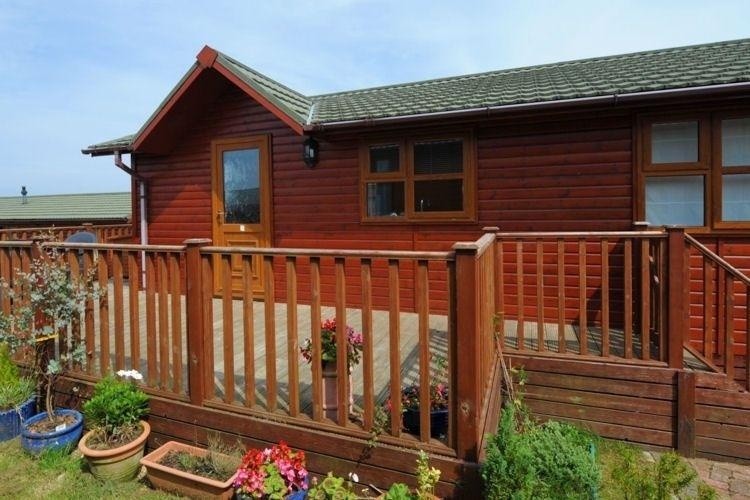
[60,230,98,276]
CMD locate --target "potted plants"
[0,334,42,445]
[138,439,245,499]
[0,223,111,458]
[477,399,603,500]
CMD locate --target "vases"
[311,364,355,420]
[401,408,450,440]
[284,472,310,499]
[77,421,152,485]
[375,488,443,500]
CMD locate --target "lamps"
[300,134,324,169]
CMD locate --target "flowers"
[378,448,445,500]
[79,368,152,445]
[229,435,310,500]
[300,318,365,366]
[380,357,449,411]
